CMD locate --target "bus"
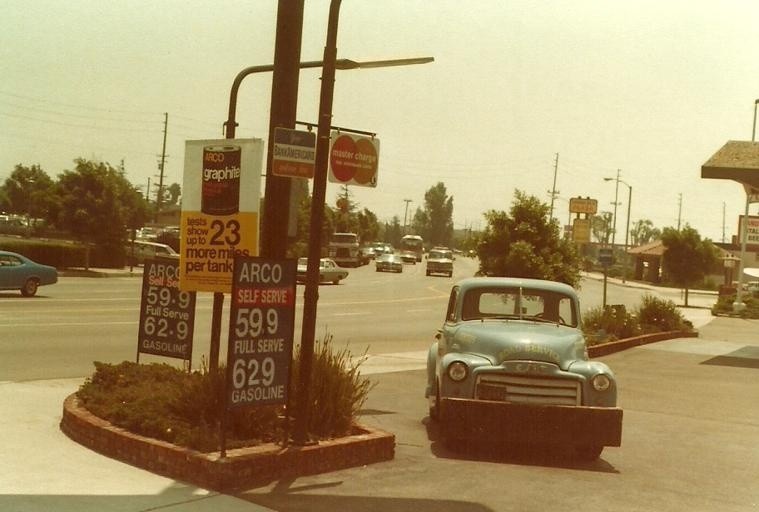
[400,234,422,262]
[330,233,360,266]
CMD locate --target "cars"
[362,241,416,273]
[1,251,57,296]
[296,258,348,285]
[423,277,623,461]
[128,227,179,266]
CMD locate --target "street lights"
[605,178,632,274]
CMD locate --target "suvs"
[425,249,455,277]
